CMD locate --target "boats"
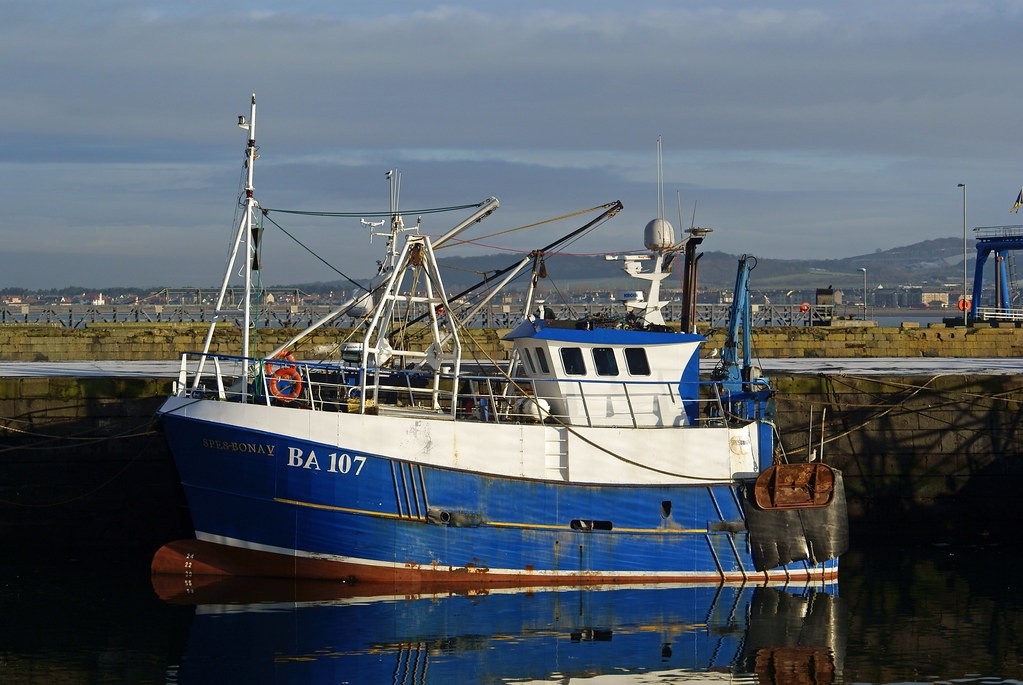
[172,587,777,685]
[149,90,846,596]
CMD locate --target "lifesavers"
[958,299,970,312]
[269,368,301,402]
[266,351,296,379]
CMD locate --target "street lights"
[957,183,967,327]
[857,268,867,320]
